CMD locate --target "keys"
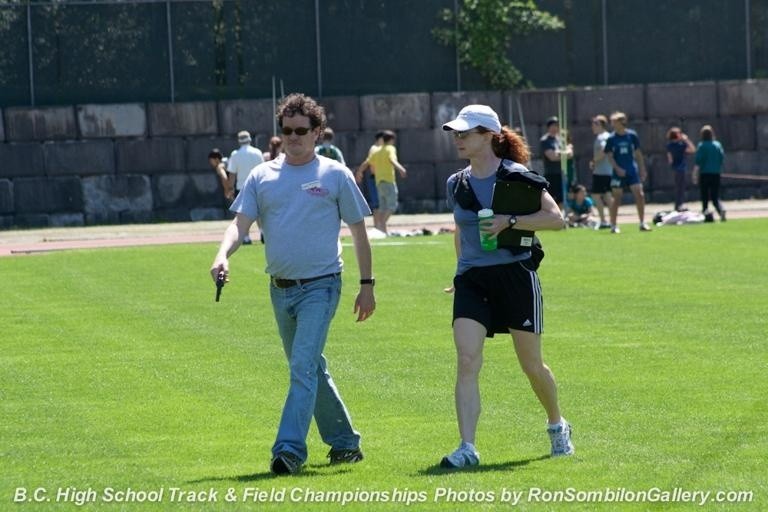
[215,277,226,303]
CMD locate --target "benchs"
[718,173,768,198]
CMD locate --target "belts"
[268,271,343,290]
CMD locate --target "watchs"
[508,215,518,229]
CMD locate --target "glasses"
[280,124,315,137]
[452,129,484,139]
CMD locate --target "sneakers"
[439,440,482,469]
[270,451,304,477]
[545,420,575,456]
[241,233,266,246]
[326,447,365,467]
[599,205,727,234]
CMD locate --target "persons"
[540,115,573,209]
[693,124,730,224]
[262,137,283,161]
[438,105,575,466]
[207,148,228,187]
[210,90,377,472]
[227,131,265,245]
[356,132,406,236]
[665,128,696,212]
[315,126,345,166]
[564,185,595,227]
[588,113,614,230]
[604,111,653,233]
[367,130,384,228]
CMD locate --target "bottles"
[477,208,497,251]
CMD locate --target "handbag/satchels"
[517,235,546,274]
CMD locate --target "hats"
[238,130,252,143]
[441,104,503,135]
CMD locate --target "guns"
[215,271,225,301]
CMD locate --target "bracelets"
[358,277,375,285]
[592,159,595,163]
